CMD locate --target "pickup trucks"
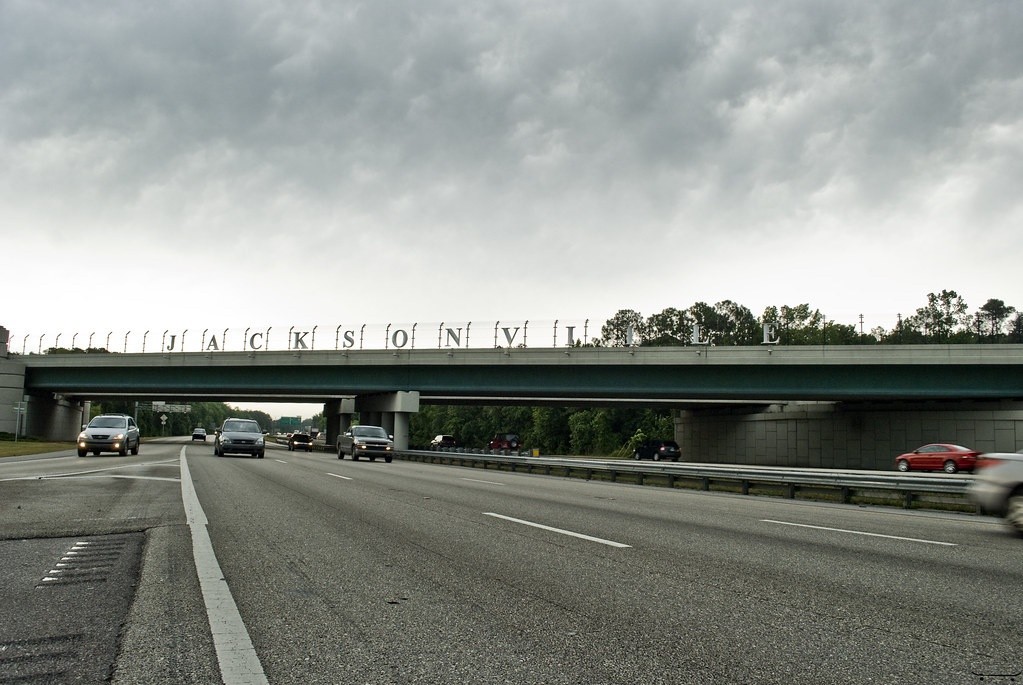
[336,426,395,463]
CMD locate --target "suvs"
[77,413,140,457]
[215,417,267,459]
[488,433,522,451]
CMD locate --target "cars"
[288,434,313,452]
[192,428,206,442]
[895,444,983,474]
[970,453,1023,538]
[316,432,326,440]
[634,439,682,462]
[431,435,456,448]
